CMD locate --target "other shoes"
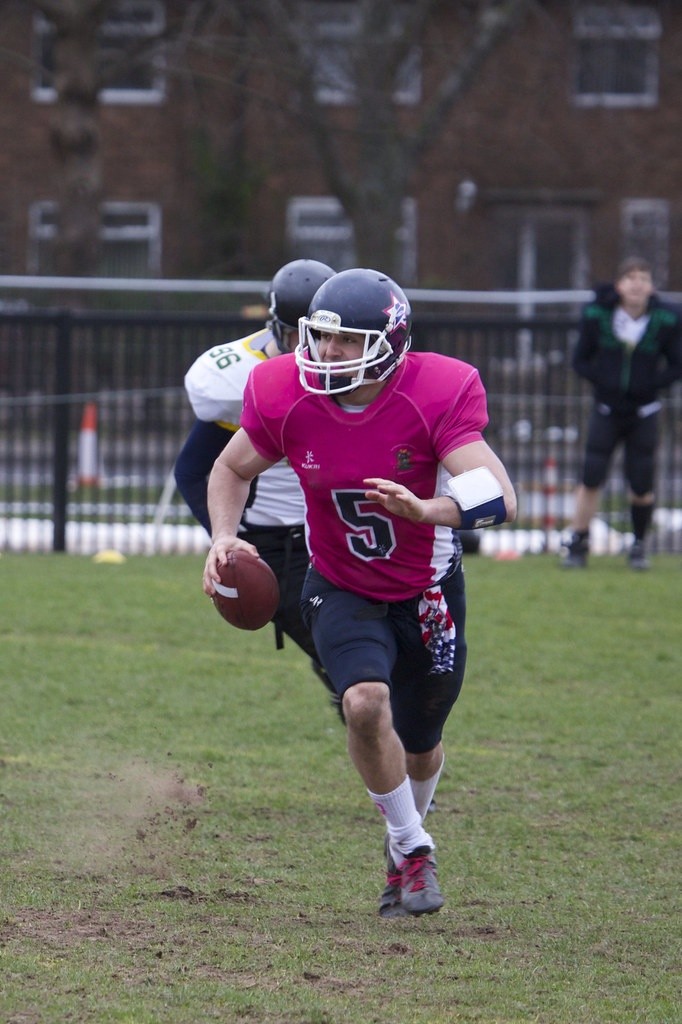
[561,527,591,568]
[628,538,649,571]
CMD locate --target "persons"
[556,256,682,570]
[175,259,517,917]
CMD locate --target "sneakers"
[398,840,444,914]
[378,831,417,921]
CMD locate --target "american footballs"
[212,550,280,632]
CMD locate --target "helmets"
[306,267,413,381]
[266,259,337,354]
